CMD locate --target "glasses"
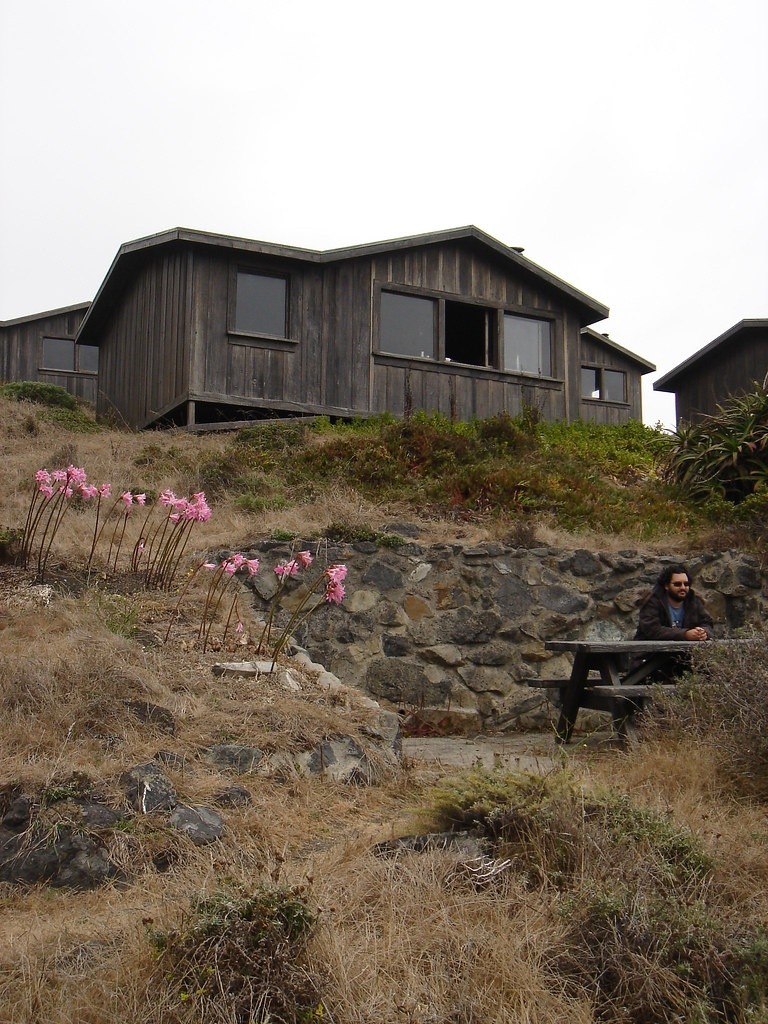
[669,581,690,587]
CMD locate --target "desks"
[545,641,752,752]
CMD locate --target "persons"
[630,567,715,684]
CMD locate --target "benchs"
[526,678,721,697]
